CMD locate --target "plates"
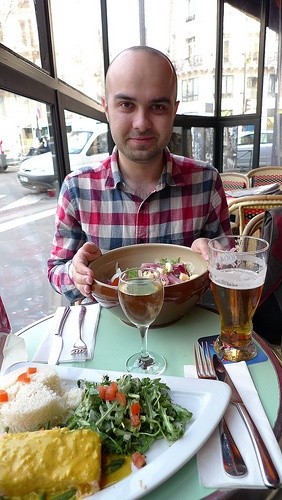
[0,362,232,500]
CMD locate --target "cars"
[0,139,8,171]
[17,123,109,196]
[204,129,273,167]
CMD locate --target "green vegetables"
[125,257,182,280]
[30,373,194,500]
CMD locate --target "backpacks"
[251,206,282,347]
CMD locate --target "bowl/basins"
[88,243,211,329]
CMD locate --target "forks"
[193,341,248,478]
[69,306,87,356]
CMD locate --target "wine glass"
[117,268,166,375]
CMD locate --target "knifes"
[211,353,281,489]
[47,306,72,365]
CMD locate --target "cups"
[208,235,269,362]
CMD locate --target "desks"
[13,300,282,500]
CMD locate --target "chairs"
[219,165,282,345]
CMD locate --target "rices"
[0,364,83,434]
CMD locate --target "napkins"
[184,361,282,490]
[32,303,101,363]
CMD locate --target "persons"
[48,45,234,312]
